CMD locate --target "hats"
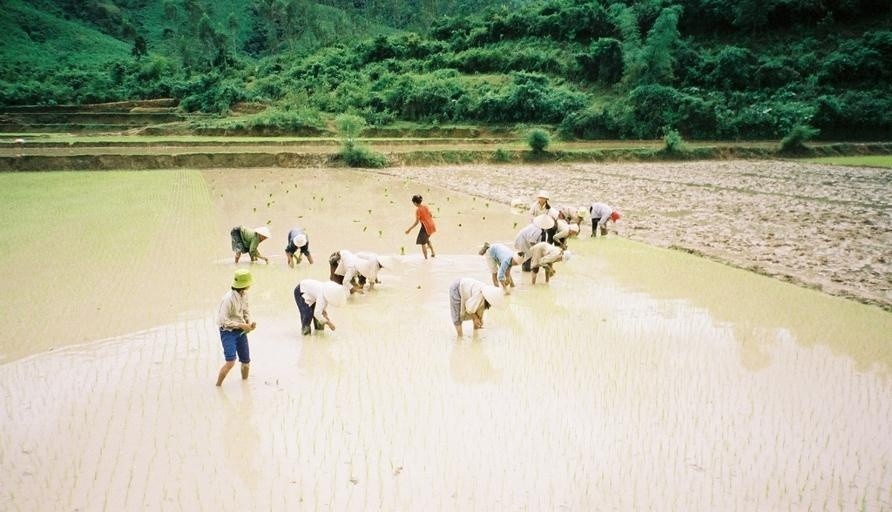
[377,254,394,269]
[611,211,621,224]
[231,269,253,289]
[354,258,378,283]
[293,235,308,247]
[533,215,556,231]
[324,279,349,308]
[578,205,589,218]
[536,190,550,199]
[483,284,506,307]
[562,250,573,264]
[254,224,271,238]
[569,223,580,232]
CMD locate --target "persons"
[406,195,436,259]
[450,189,621,337]
[216,223,386,387]
